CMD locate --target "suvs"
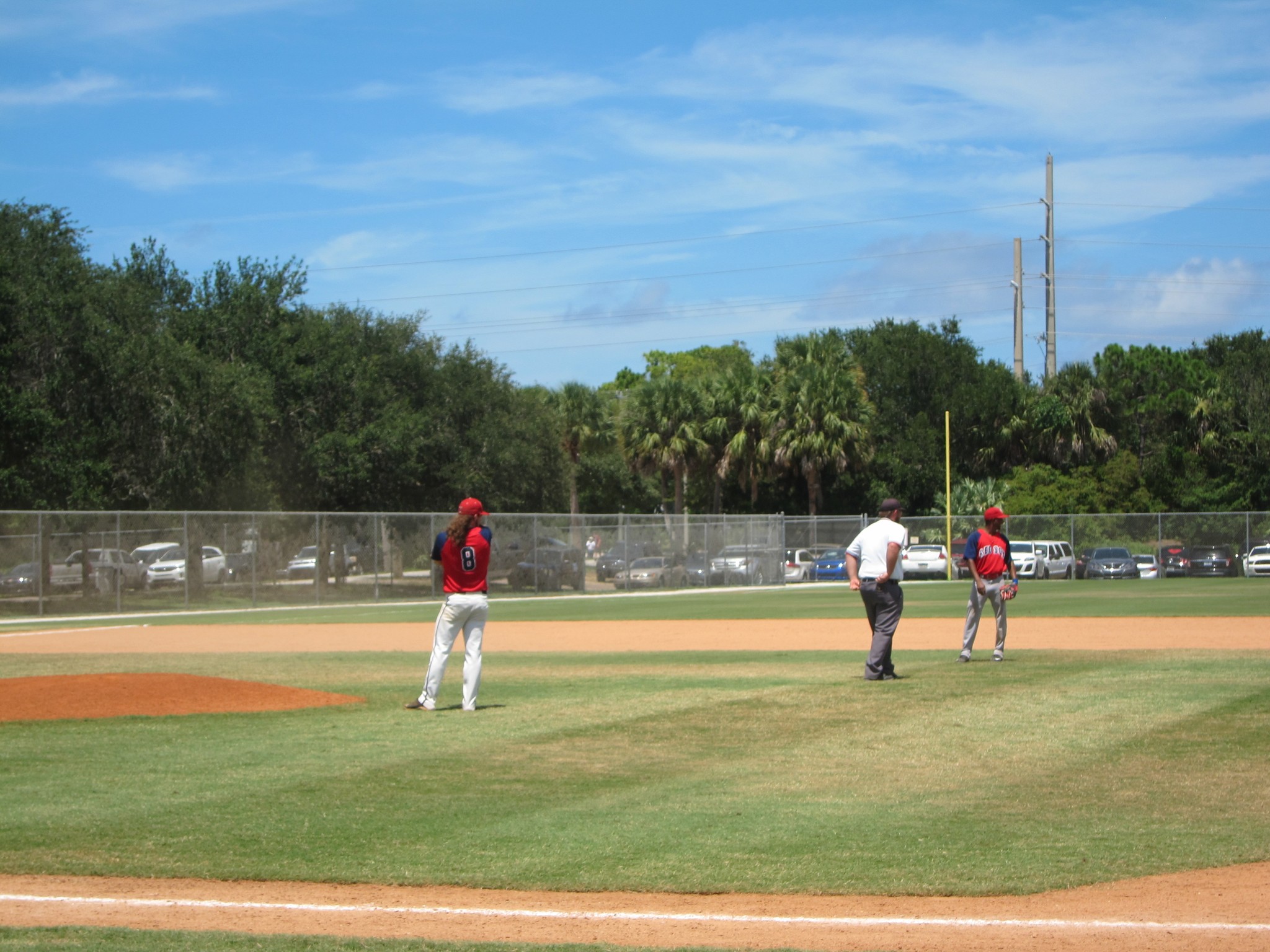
[1156,544,1191,578]
[508,545,587,593]
[708,542,770,585]
[596,542,644,583]
[1188,542,1238,577]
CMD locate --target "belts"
[979,572,1003,579]
[456,589,487,594]
[862,578,898,583]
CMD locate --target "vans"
[1036,539,1077,579]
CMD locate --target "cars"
[945,538,972,576]
[1130,553,1161,579]
[145,546,228,590]
[1009,540,1045,580]
[780,548,814,583]
[285,543,349,580]
[901,544,961,581]
[0,562,42,597]
[612,556,690,589]
[1083,547,1140,579]
[810,547,861,581]
[1241,545,1270,577]
[224,551,277,585]
[1077,548,1094,580]
[130,542,181,567]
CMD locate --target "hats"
[879,499,905,511]
[458,497,489,515]
[984,507,1009,520]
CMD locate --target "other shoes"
[993,655,1003,660]
[957,655,969,662]
[877,672,898,680]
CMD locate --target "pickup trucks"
[49,548,147,595]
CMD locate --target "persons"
[846,499,906,681]
[586,531,602,558]
[405,496,491,709]
[958,508,1019,661]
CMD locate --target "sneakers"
[406,699,428,712]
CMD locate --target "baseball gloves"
[1000,583,1018,601]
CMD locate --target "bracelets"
[1012,579,1018,585]
